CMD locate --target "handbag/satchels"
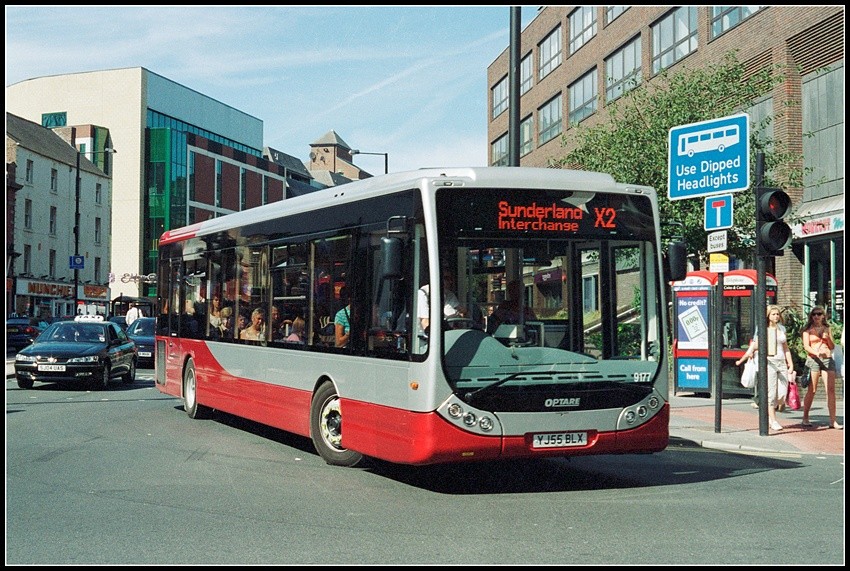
[786,370,801,410]
[739,356,756,387]
[801,365,812,387]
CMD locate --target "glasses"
[443,275,452,280]
[812,313,823,316]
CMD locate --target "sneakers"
[769,414,772,425]
[771,420,783,430]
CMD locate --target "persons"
[488,280,540,346]
[162,272,392,351]
[802,306,844,431]
[125,300,144,327]
[735,305,797,431]
[413,261,468,348]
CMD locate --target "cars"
[13,315,138,388]
[126,318,155,366]
[6,317,49,350]
[110,315,127,330]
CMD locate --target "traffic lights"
[755,186,791,257]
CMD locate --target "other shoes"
[802,419,812,425]
[829,423,844,429]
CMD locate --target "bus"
[153,167,688,468]
